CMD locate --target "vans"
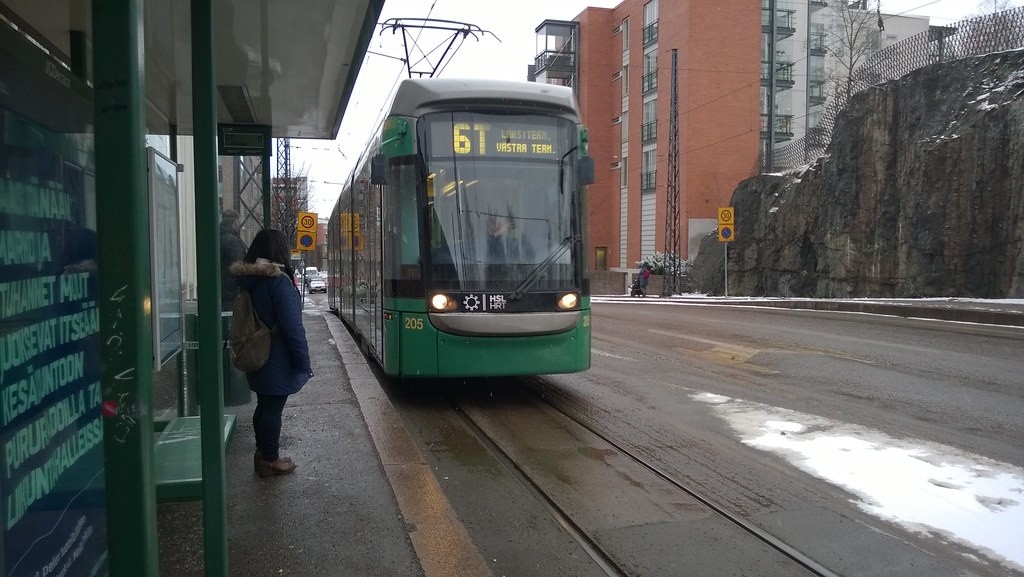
[305,267,318,279]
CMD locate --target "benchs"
[151,415,236,503]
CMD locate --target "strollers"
[631,276,643,297]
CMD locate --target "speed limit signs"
[301,216,314,228]
[721,210,732,221]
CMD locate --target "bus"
[328,80,592,376]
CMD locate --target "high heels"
[255,450,296,477]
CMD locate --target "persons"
[627,261,648,297]
[219,209,249,311]
[229,229,314,479]
[488,212,513,239]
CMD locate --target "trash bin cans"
[223,311,252,407]
[159,313,198,414]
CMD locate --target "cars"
[308,279,326,293]
[318,271,328,278]
[306,276,324,287]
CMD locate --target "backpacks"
[229,290,272,374]
